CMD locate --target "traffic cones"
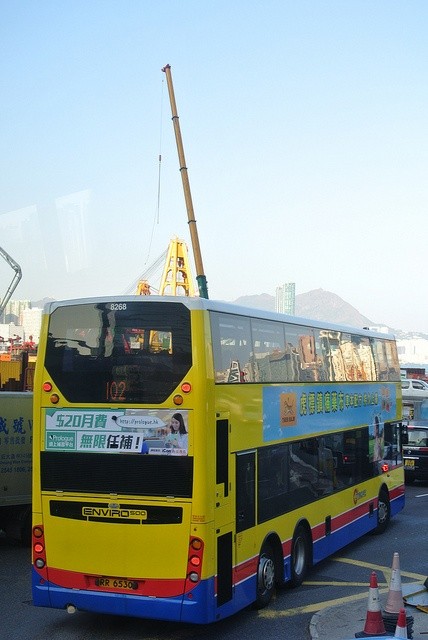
[395,607,407,639]
[355,572,394,638]
[382,552,414,639]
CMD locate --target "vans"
[401,379,428,400]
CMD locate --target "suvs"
[401,426,428,485]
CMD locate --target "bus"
[32,295,405,625]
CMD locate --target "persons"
[162,413,189,450]
